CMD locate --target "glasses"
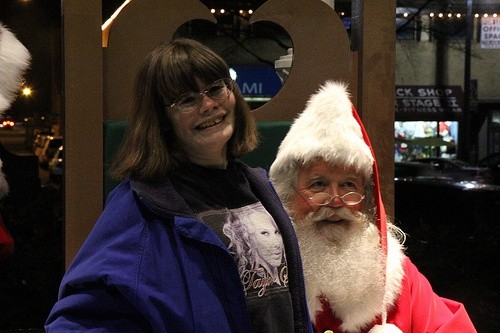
[295,187,367,206]
[170,79,233,114]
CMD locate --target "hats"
[270,80,374,180]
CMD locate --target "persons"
[44,38,313,333]
[268,79,477,333]
